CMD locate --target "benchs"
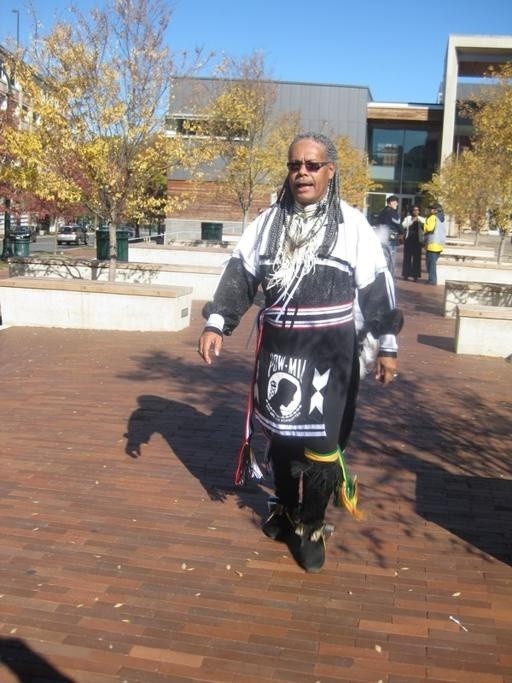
[0,231,242,331]
[434,236,512,359]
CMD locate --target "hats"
[427,200,442,209]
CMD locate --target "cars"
[79,222,95,233]
[6,224,37,243]
[96,221,134,239]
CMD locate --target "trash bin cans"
[95,229,130,262]
[201,221,223,241]
[13,239,29,256]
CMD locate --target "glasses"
[284,158,334,171]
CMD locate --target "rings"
[393,372,397,377]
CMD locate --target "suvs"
[55,225,90,246]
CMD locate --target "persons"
[421,203,446,285]
[195,130,406,573]
[376,194,408,278]
[400,204,427,282]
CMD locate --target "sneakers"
[298,523,330,574]
[264,498,302,538]
